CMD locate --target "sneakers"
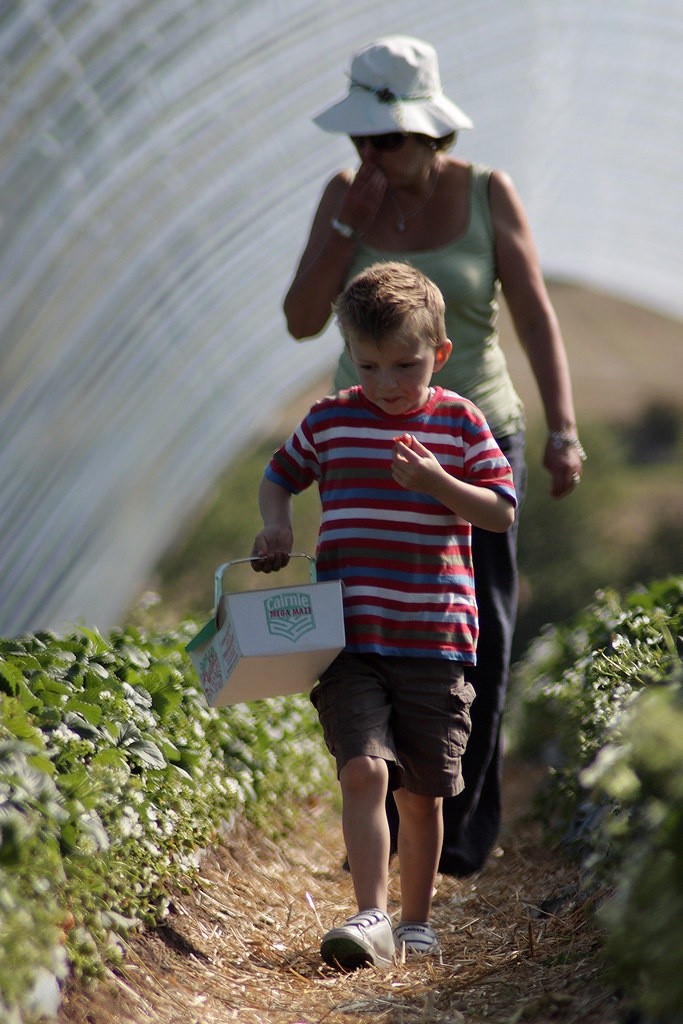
[321,908,395,968]
[393,921,440,955]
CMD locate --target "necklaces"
[388,160,441,232]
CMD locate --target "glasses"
[351,132,409,154]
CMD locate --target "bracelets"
[327,215,366,240]
[544,431,586,460]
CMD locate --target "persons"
[250,262,517,971]
[283,35,586,899]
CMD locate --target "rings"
[572,473,581,484]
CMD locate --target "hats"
[312,36,473,138]
